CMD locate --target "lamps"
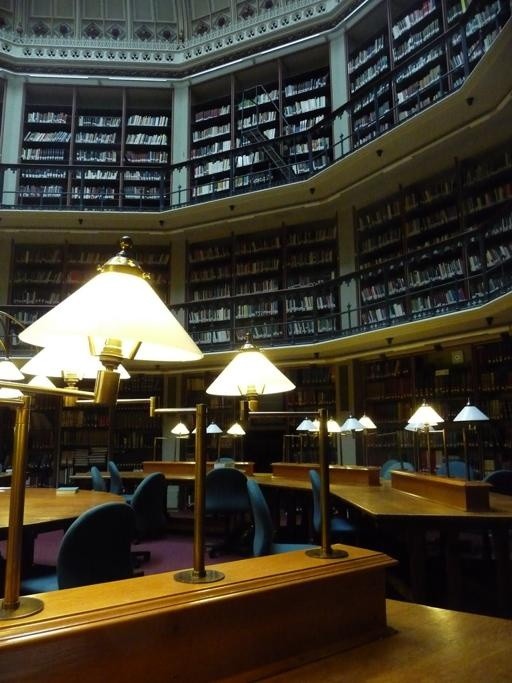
[399,398,486,479]
[296,413,378,463]
[173,420,248,448]
[206,341,348,560]
[1,237,208,616]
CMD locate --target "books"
[347,33,393,150]
[0,376,163,494]
[189,223,337,344]
[391,1,447,121]
[445,1,502,88]
[362,341,512,481]
[191,75,329,198]
[355,146,512,330]
[186,368,337,465]
[9,250,170,348]
[20,112,168,199]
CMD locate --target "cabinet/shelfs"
[3,375,159,482]
[344,3,512,149]
[189,71,333,201]
[416,346,510,462]
[181,375,232,450]
[356,139,509,334]
[24,92,169,209]
[8,239,172,349]
[361,353,414,455]
[289,368,333,455]
[187,211,340,351]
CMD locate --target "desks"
[2,466,508,683]
